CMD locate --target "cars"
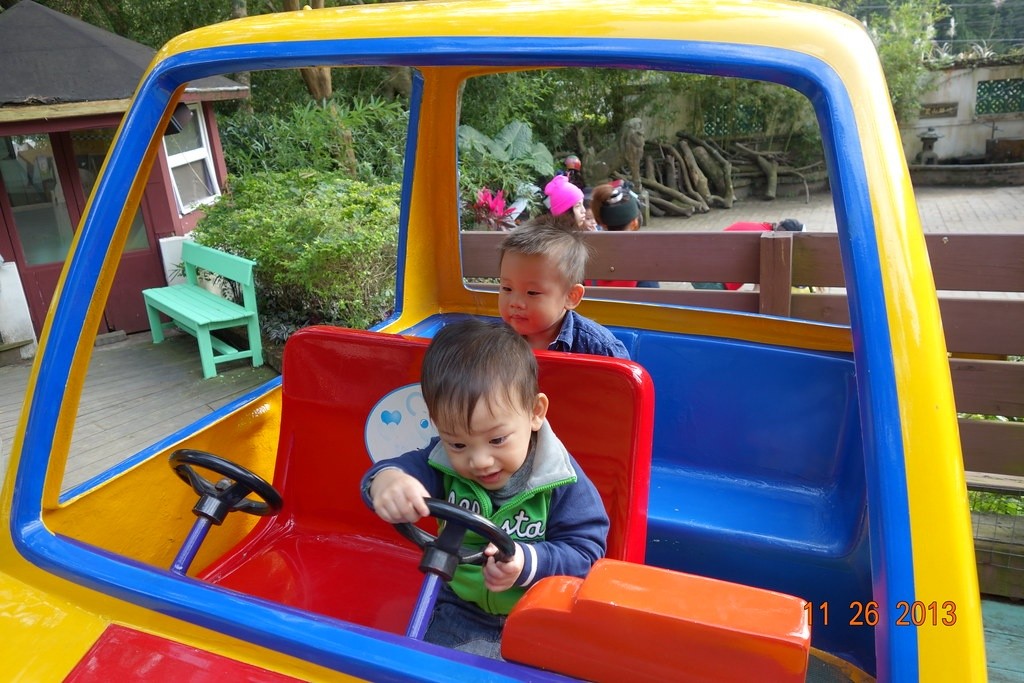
[0,1,990,683]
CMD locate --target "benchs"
[191,325,654,636]
[141,238,265,379]
[392,311,878,682]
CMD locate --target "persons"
[537,174,660,288]
[498,214,630,359]
[691,218,803,290]
[360,317,609,661]
